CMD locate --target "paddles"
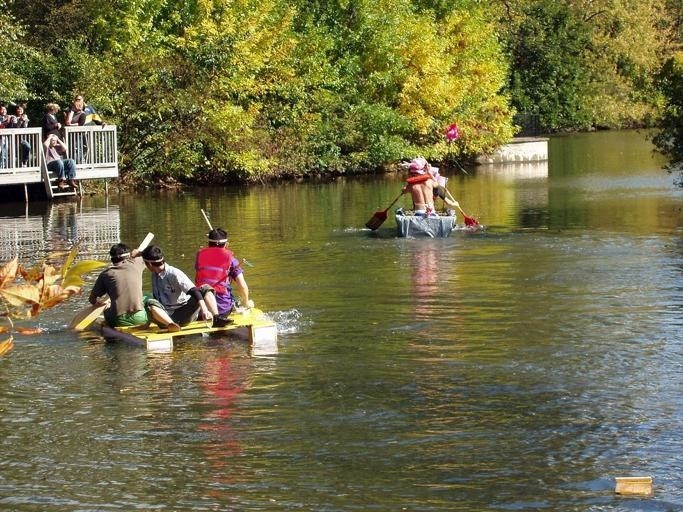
[72,231,155,330]
[444,187,478,226]
[365,183,409,230]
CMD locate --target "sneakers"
[214,314,234,326]
[169,321,179,331]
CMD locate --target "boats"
[394,208,457,239]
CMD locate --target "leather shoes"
[67,181,79,189]
[58,182,67,192]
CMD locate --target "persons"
[433,186,461,208]
[141,246,235,335]
[400,163,440,216]
[87,243,149,328]
[0,95,108,190]
[194,228,257,319]
